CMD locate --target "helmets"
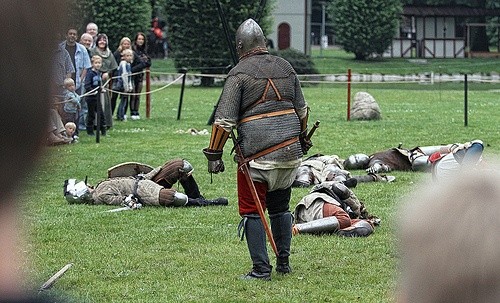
[64,179,89,203]
[235,19,268,58]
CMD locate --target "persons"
[43,22,153,144]
[201,18,319,278]
[64,159,230,206]
[148,15,170,61]
[0,0,78,303]
[394,158,500,303]
[291,171,378,236]
[344,140,484,183]
[297,152,357,188]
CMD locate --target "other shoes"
[131,112,139,116]
[57,134,73,142]
[214,197,228,206]
[47,135,64,142]
[276,262,291,273]
[240,271,272,282]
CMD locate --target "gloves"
[202,149,224,174]
[122,194,140,209]
[300,136,313,154]
